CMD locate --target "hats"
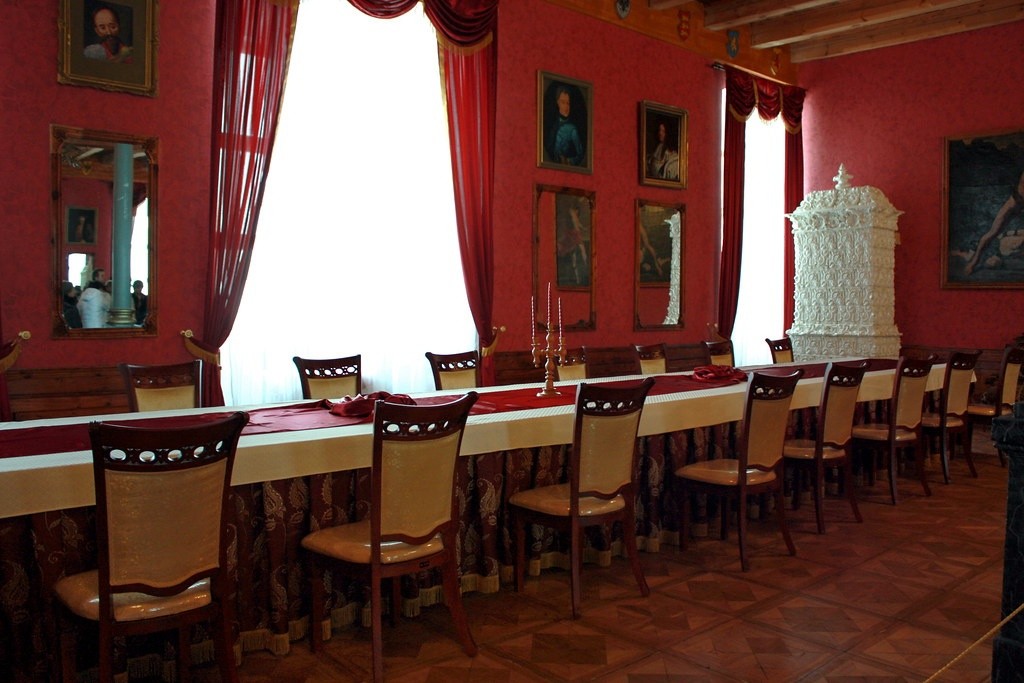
[61,280,72,293]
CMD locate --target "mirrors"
[48,122,160,339]
[65,250,95,292]
[632,197,686,331]
[531,182,598,332]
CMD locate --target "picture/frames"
[536,69,595,175]
[56,0,160,98]
[551,192,590,291]
[64,204,97,246]
[640,98,689,191]
[939,126,1023,289]
[639,204,673,287]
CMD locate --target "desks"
[0,356,977,682]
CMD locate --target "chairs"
[50,410,251,683]
[630,341,668,374]
[676,341,1024,573]
[426,350,481,390]
[509,377,653,623]
[299,391,478,683]
[700,338,735,368]
[554,357,587,380]
[118,358,203,411]
[292,354,361,399]
[765,337,793,364]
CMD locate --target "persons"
[63,268,149,330]
